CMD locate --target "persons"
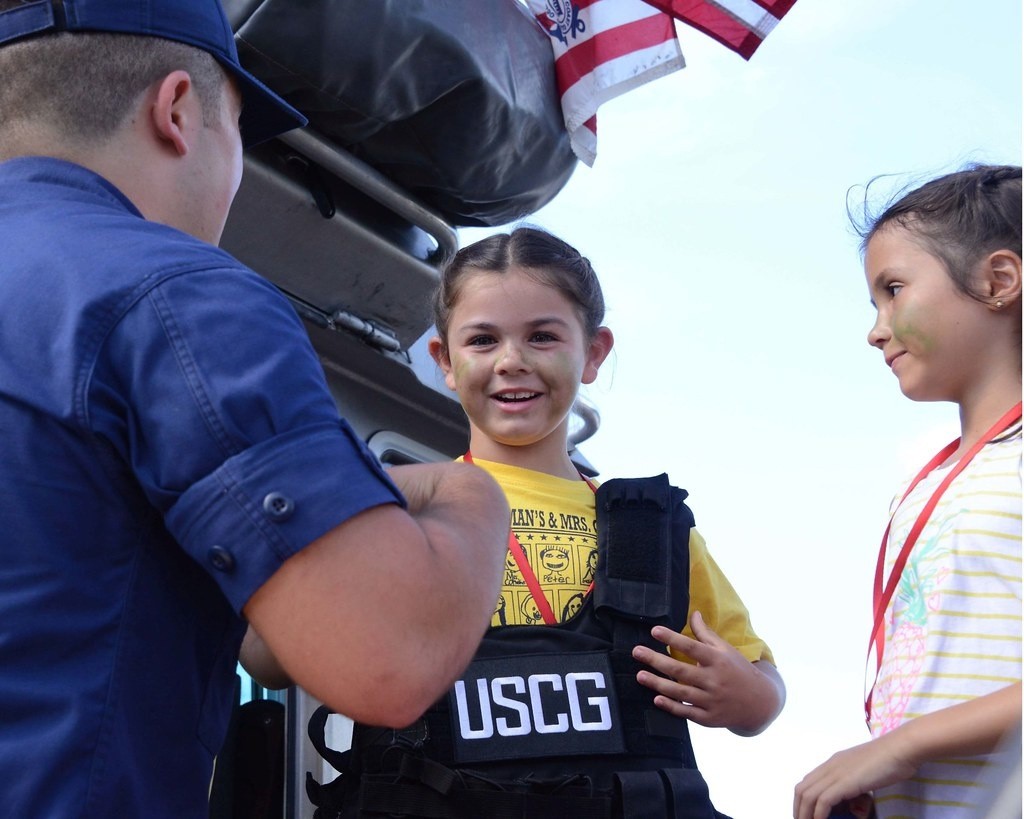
[307,228,785,819]
[0,0,511,819]
[794,165,1022,819]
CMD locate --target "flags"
[527,0,797,167]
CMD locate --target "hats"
[0,0,308,154]
